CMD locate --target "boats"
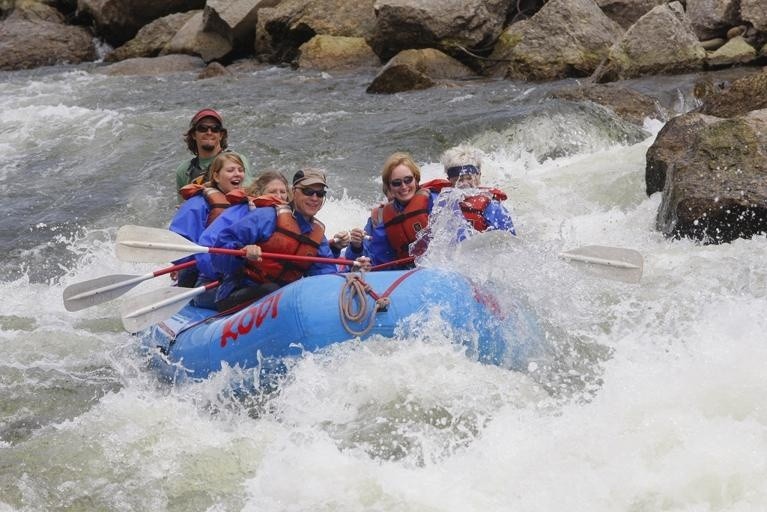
[135,266,541,409]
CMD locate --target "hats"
[191,108,223,124]
[293,168,329,188]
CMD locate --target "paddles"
[63,260,197,313]
[114,224,371,273]
[119,233,351,333]
[358,229,521,272]
[555,245,644,285]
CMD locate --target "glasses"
[197,125,220,132]
[389,175,413,186]
[295,187,326,197]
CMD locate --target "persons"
[344,152,466,272]
[193,171,291,310]
[175,108,250,212]
[434,145,515,238]
[210,167,372,314]
[167,150,246,287]
